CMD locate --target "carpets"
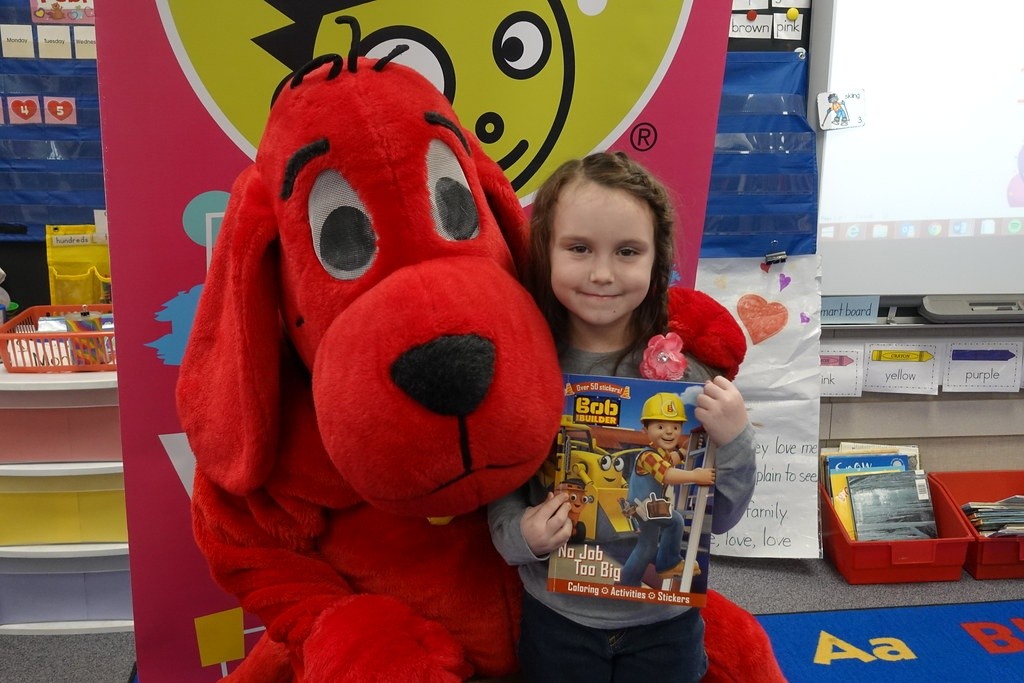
[750,597,1024,682]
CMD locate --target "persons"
[488,151,757,683]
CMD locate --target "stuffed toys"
[175,15,784,683]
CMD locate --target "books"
[821,441,938,541]
[961,494,1024,537]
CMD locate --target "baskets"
[0,304,119,374]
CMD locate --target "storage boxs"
[818,473,975,584]
[929,469,1024,579]
[0,303,118,373]
